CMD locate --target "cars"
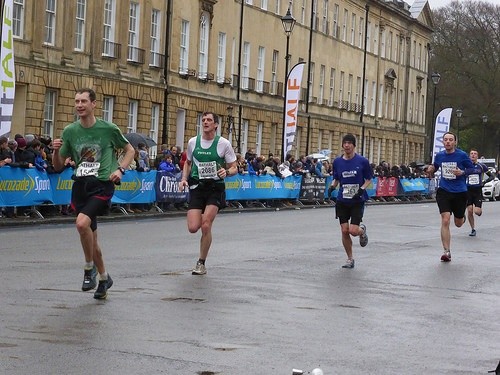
[481,177,500,201]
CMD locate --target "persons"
[365,161,438,202]
[53,88,135,299]
[328,135,374,268]
[466,148,495,236]
[424,132,472,261]
[0,133,74,219]
[111,144,190,214]
[227,149,336,207]
[497,171,500,180]
[178,112,238,275]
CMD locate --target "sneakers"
[469,229,476,236]
[341,258,355,268]
[440,252,451,261]
[359,224,368,247]
[81,264,98,291]
[93,272,113,298]
[191,258,207,275]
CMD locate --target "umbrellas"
[320,150,332,155]
[410,161,424,167]
[124,133,157,149]
[307,153,326,159]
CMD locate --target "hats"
[8,140,18,149]
[15,134,23,141]
[341,134,356,148]
[17,138,26,147]
[25,134,35,143]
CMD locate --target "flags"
[432,108,452,188]
[0,0,15,135]
[282,62,306,162]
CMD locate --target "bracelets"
[226,171,229,175]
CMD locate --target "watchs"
[118,167,125,175]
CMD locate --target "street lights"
[280,7,298,164]
[429,69,441,164]
[456,107,463,147]
[482,115,489,158]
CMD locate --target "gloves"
[328,186,336,197]
[352,187,365,201]
